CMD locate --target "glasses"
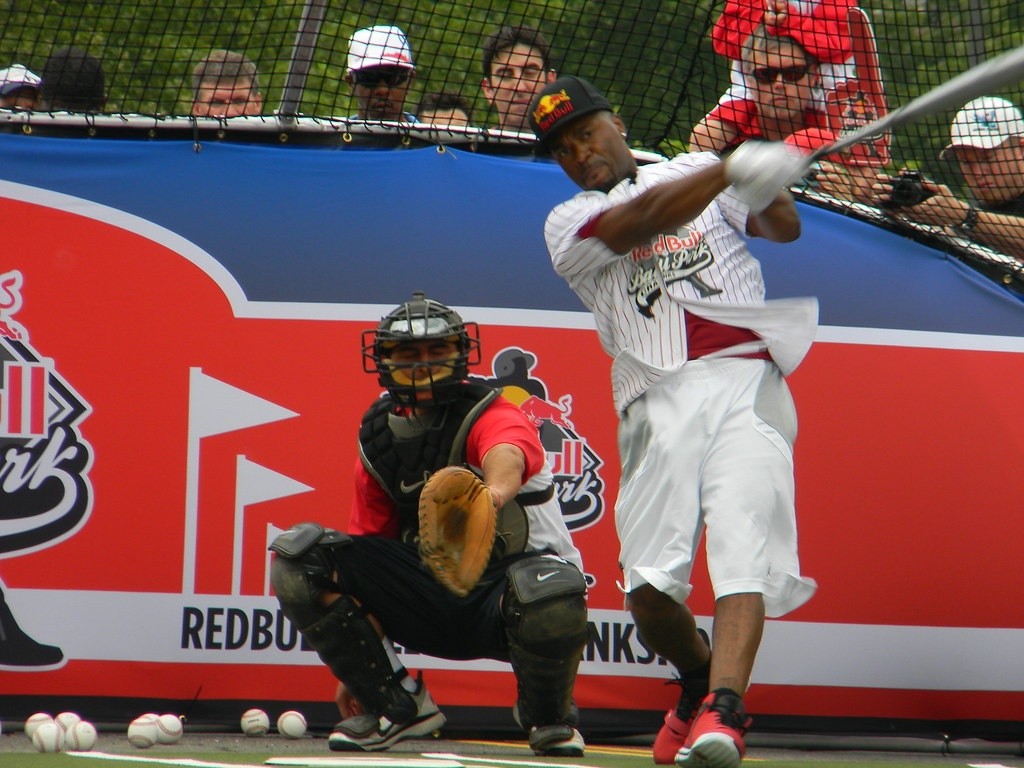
[355,68,408,87]
[752,64,809,84]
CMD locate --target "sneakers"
[653,673,708,765]
[674,687,753,768]
[513,697,585,757]
[328,669,448,752]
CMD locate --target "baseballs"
[55,713,81,733]
[241,709,270,738]
[156,714,182,744]
[140,714,159,722]
[65,721,96,752]
[127,718,157,748]
[33,723,65,753]
[277,711,307,739]
[24,713,54,742]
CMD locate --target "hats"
[940,96,1024,159]
[529,75,613,144]
[346,25,416,72]
[0,63,42,95]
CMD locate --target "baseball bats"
[805,44,1024,168]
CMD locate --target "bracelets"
[957,205,979,232]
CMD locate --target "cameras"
[882,171,934,210]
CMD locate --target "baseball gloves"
[418,466,497,597]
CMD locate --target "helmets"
[361,290,481,431]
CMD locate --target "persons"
[687,0,890,208]
[191,47,264,118]
[0,63,42,113]
[868,90,1024,267]
[481,24,557,135]
[417,91,470,127]
[37,45,108,113]
[341,23,422,124]
[267,294,592,759]
[529,79,820,768]
